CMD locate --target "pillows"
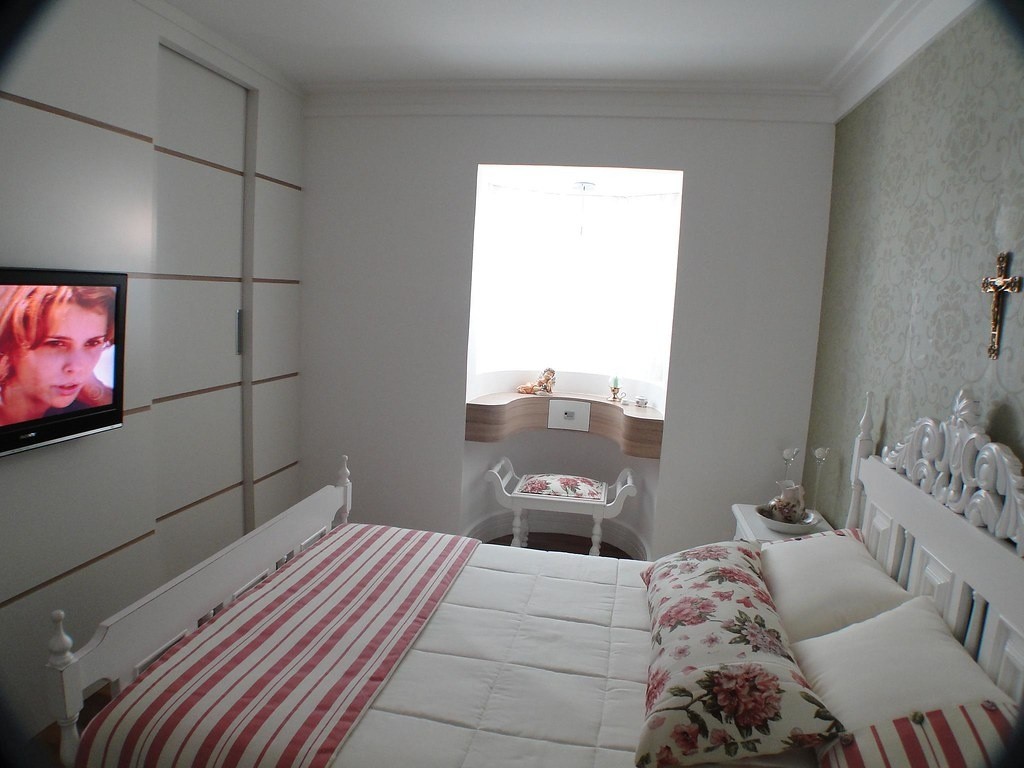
[634,538,846,768]
[791,596,1024,768]
[760,528,912,641]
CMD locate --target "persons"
[533,368,556,392]
[0,284,116,426]
[987,283,1010,328]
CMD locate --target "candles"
[613,376,619,387]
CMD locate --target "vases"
[767,480,804,524]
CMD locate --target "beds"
[47,387,1024,768]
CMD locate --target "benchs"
[485,456,637,557]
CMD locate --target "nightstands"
[731,503,835,542]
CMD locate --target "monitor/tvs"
[0,266,128,457]
